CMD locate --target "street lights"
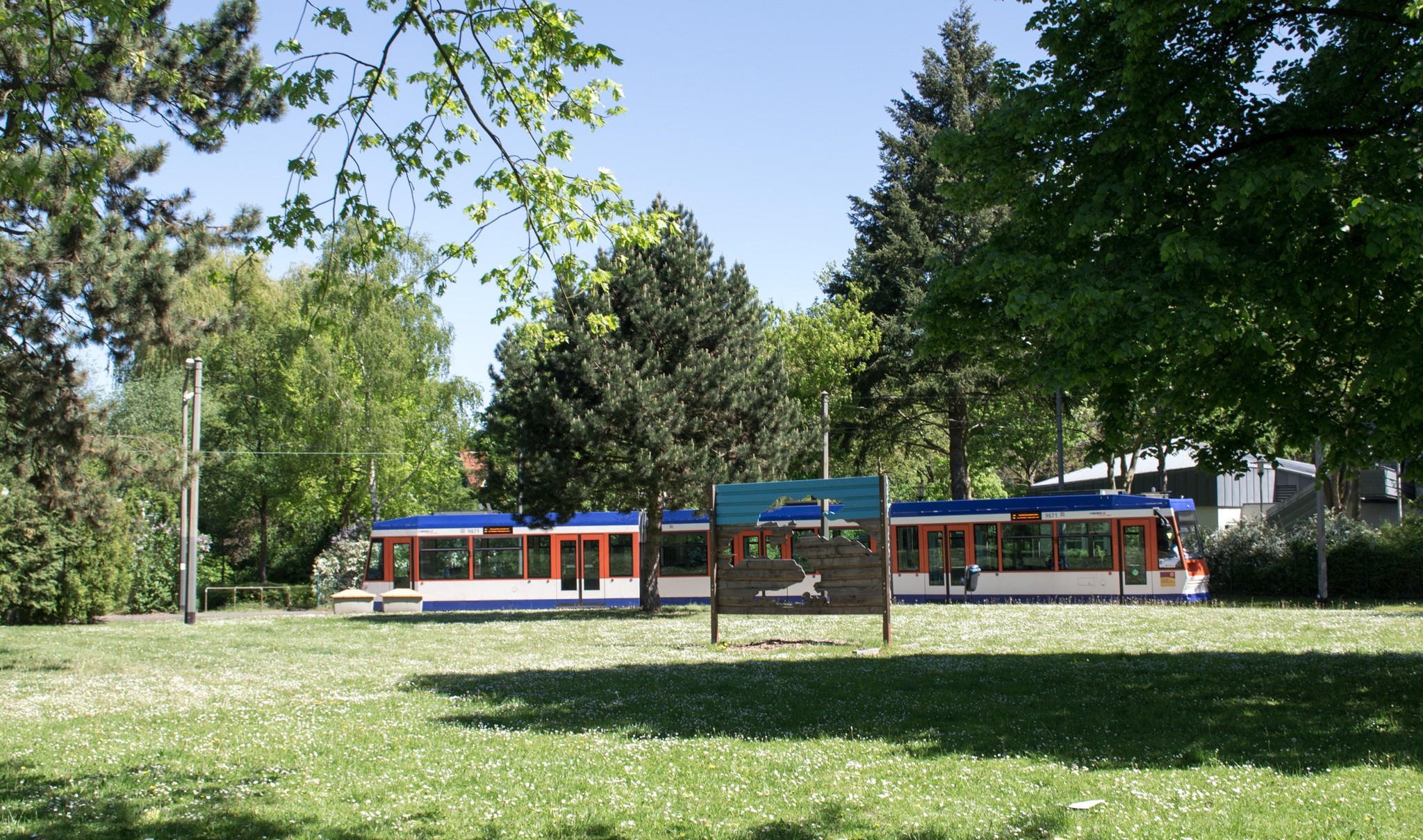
[1251,458,1271,521]
[178,359,197,613]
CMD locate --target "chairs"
[1010,546,1113,570]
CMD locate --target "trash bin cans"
[380,589,424,612]
[966,563,981,592]
[330,588,377,616]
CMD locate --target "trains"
[359,493,1211,615]
